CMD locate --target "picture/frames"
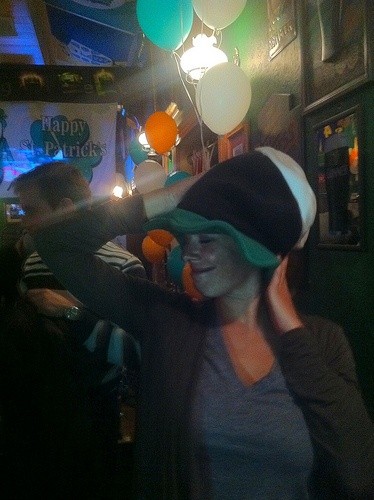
[227,120,249,160]
[311,103,369,252]
[295,0,370,118]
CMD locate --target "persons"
[30,146,374,500]
[0,163,141,500]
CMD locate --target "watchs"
[63,304,79,323]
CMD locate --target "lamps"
[180,0,228,84]
[138,130,155,156]
[109,182,129,202]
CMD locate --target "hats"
[142,146,317,278]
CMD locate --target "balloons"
[136,0,270,136]
[129,113,190,196]
